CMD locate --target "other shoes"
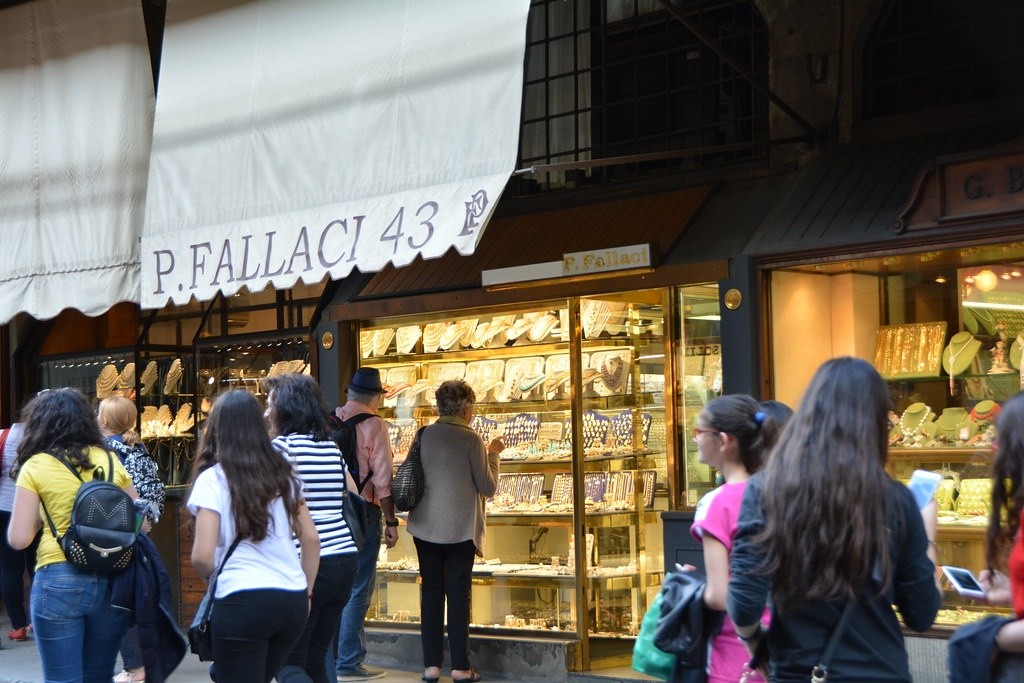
[421,666,442,683]
[8,626,27,641]
[28,624,34,632]
[336,662,387,682]
[452,668,480,683]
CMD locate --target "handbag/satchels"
[344,493,383,551]
[187,591,214,662]
[631,570,675,680]
[391,425,427,511]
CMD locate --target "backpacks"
[326,410,382,494]
[36,446,136,576]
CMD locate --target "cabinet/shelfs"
[351,285,678,672]
[875,373,1024,616]
[39,343,199,497]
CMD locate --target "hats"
[346,367,388,397]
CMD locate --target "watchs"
[386,518,399,526]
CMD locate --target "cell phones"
[908,469,940,510]
[941,566,985,599]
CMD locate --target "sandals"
[112,665,146,683]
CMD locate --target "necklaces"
[360,299,628,402]
[948,335,973,395]
[970,307,993,322]
[96,362,213,473]
[268,360,310,377]
[885,400,1001,437]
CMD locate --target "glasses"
[94,410,109,426]
[692,423,736,444]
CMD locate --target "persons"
[330,366,399,682]
[0,387,166,683]
[674,393,795,683]
[262,373,360,683]
[186,388,321,683]
[952,390,1024,683]
[406,378,507,683]
[724,356,944,683]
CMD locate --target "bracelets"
[308,592,313,598]
[737,624,762,643]
[928,539,938,553]
